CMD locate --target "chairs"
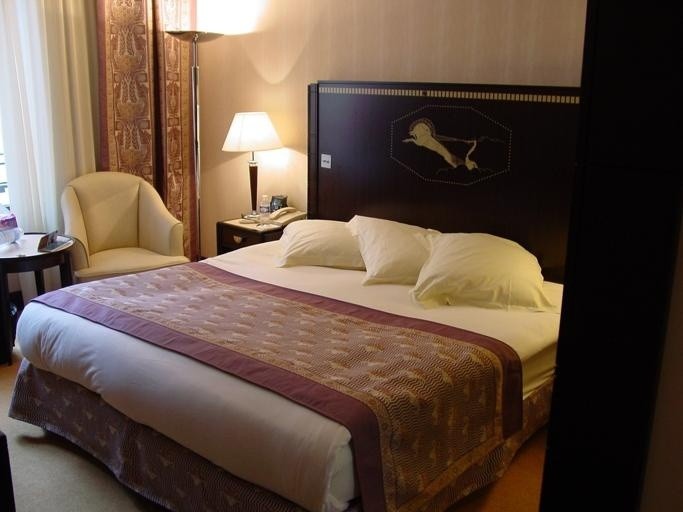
[60,170,191,285]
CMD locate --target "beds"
[15,78,586,512]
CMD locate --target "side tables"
[1,232,76,367]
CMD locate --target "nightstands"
[215,218,282,255]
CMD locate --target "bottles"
[260,195,271,228]
[270,195,288,214]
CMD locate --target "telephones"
[267,207,307,227]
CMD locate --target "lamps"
[220,111,283,224]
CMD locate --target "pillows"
[272,211,555,316]
